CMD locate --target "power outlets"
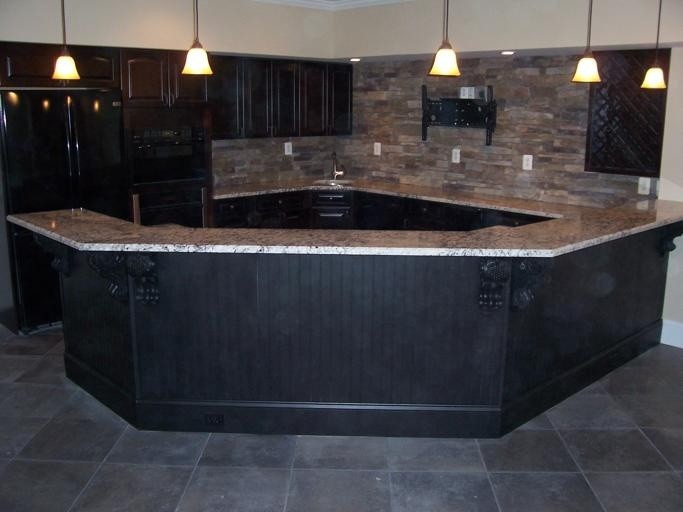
[451,149,460,164]
[521,155,532,171]
[373,143,381,156]
[637,176,650,195]
[283,142,293,155]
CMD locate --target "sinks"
[313,178,353,185]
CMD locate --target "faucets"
[330,151,347,180]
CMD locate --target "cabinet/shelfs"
[0,48,130,335]
[207,54,352,141]
[217,187,565,232]
[117,47,209,107]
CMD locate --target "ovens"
[312,193,354,230]
[123,107,215,229]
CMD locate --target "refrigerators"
[0,86,122,338]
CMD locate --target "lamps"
[50,1,81,81]
[179,1,213,76]
[570,1,603,84]
[427,0,461,78]
[636,1,668,89]
